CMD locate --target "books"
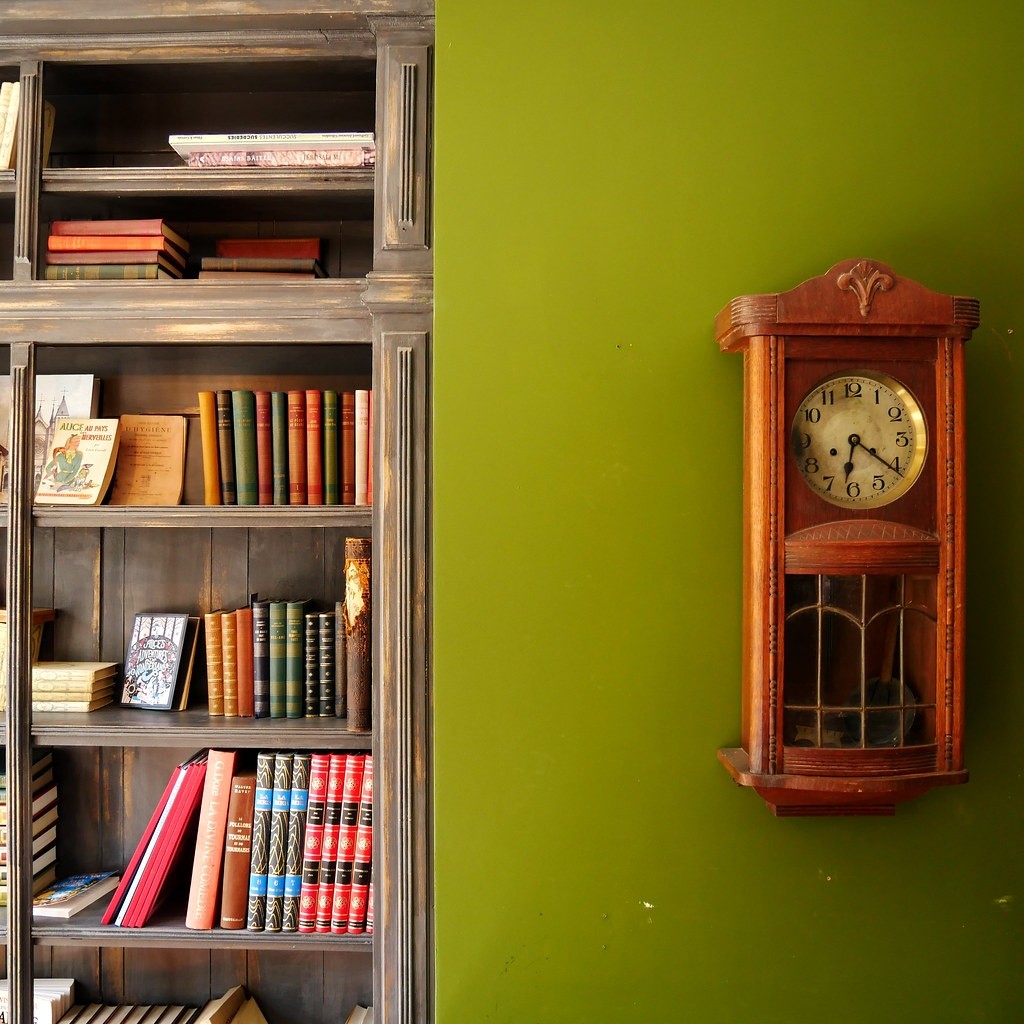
[1,375,373,510]
[0,538,372,734]
[0,978,374,1024]
[0,750,373,934]
[0,80,376,280]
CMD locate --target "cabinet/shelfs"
[0,0,436,1024]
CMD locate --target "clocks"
[716,258,983,816]
